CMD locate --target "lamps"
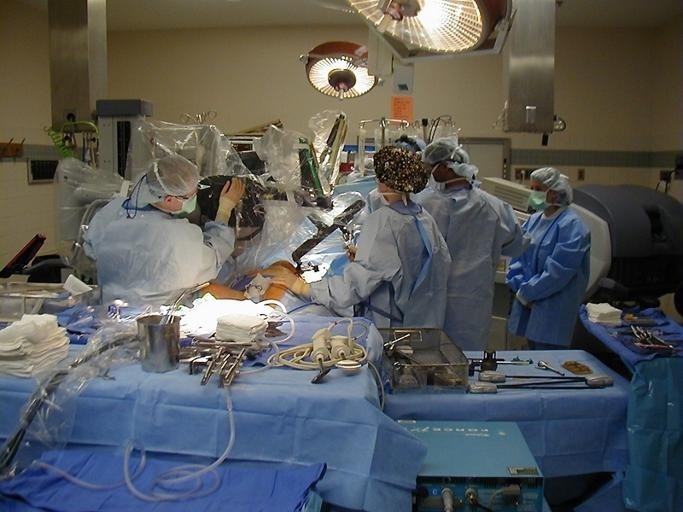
[303,42,380,101]
[352,0,510,56]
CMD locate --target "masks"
[377,189,391,206]
[528,191,550,209]
[181,194,197,214]
[427,173,447,194]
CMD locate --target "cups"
[136,315,179,373]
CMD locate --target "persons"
[266,142,453,330]
[345,130,435,245]
[83,153,248,312]
[411,135,531,351]
[504,167,593,352]
